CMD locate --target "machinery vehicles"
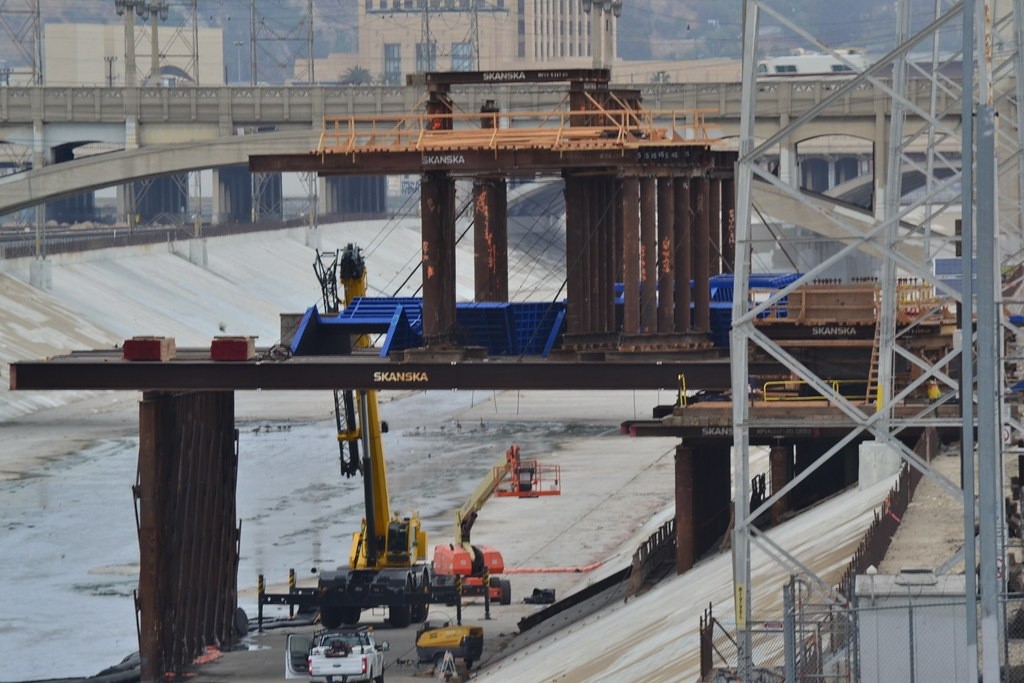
[424,443,560,606]
[252,242,490,627]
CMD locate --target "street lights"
[602,0,623,70]
[114,0,146,118]
[582,0,612,70]
[142,0,170,87]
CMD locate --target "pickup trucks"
[285,627,390,683]
